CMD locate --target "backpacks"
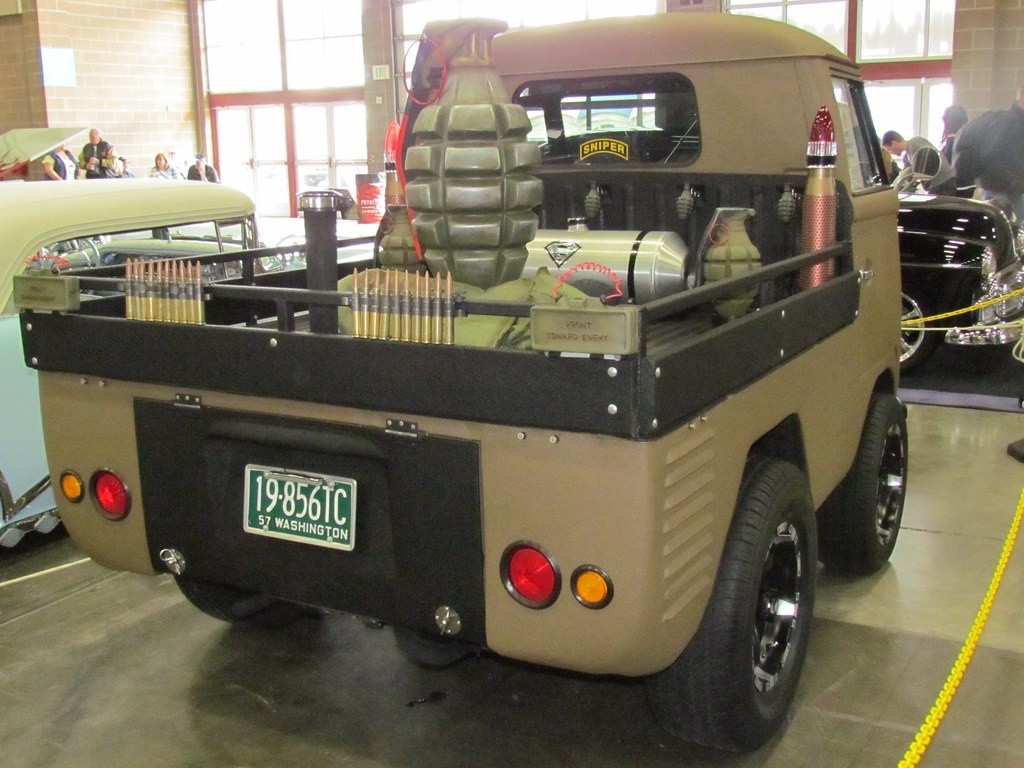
[952,109,1018,179]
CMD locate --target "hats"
[117,157,131,163]
[194,154,205,162]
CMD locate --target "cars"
[899,189,1024,375]
[1,175,378,550]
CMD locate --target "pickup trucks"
[11,9,912,753]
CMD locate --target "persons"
[79,129,118,179]
[44,143,82,180]
[979,87,1024,223]
[187,156,219,183]
[115,157,136,178]
[940,104,977,198]
[883,131,958,196]
[149,153,179,179]
